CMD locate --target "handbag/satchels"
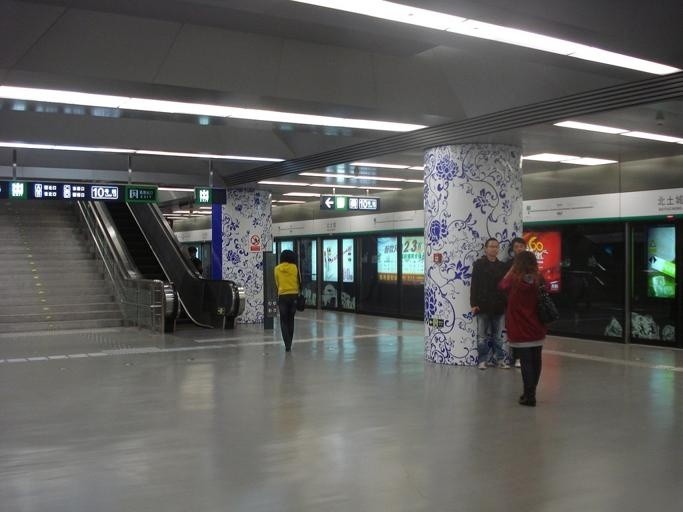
[297,295,306,312]
[537,274,558,323]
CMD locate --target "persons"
[502,238,527,369]
[186,246,201,270]
[470,237,510,370]
[274,250,302,352]
[498,251,547,406]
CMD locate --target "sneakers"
[478,359,521,369]
